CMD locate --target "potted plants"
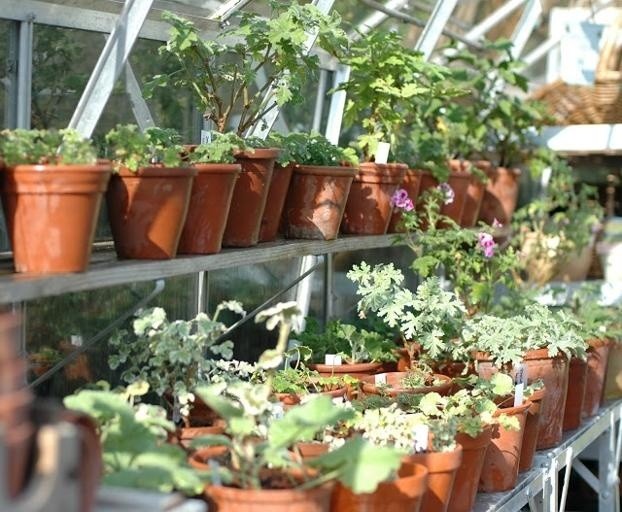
[103,120,194,262]
[1,123,112,277]
[68,259,622,512]
[141,0,537,255]
